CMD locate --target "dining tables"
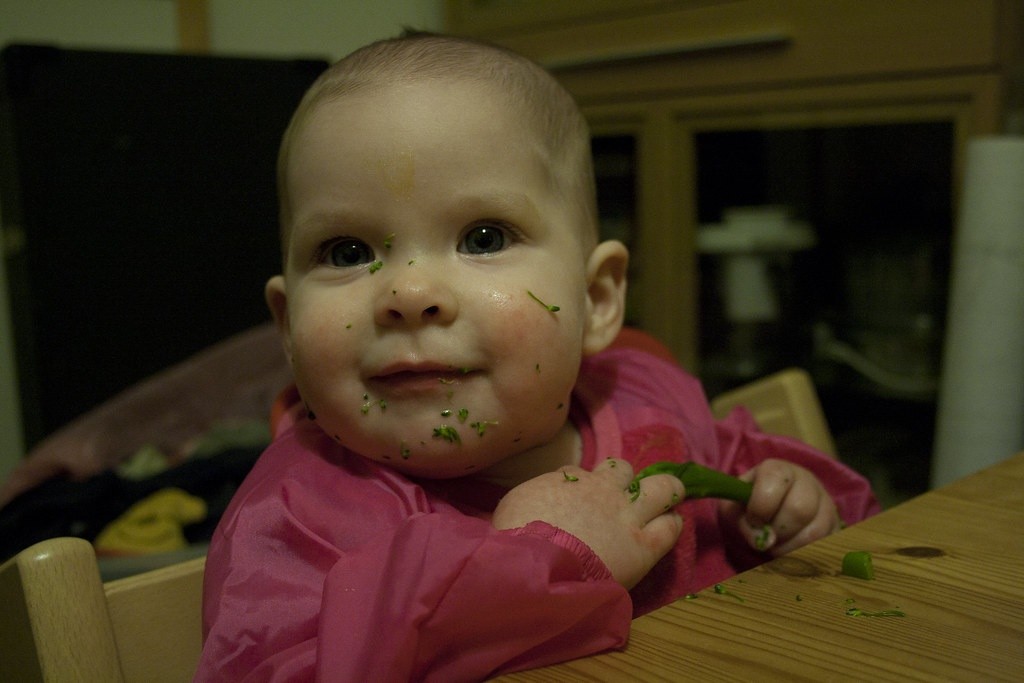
[482,444,1024,681]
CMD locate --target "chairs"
[0,371,848,682]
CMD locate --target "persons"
[193,26,872,683]
